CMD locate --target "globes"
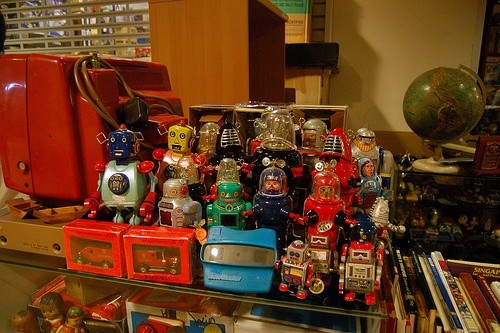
[403,63,485,174]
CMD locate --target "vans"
[76,245,113,269]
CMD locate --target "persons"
[11,294,63,333]
[81,109,405,307]
[58,308,87,333]
[361,159,376,178]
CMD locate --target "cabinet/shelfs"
[391,168,500,265]
[148,0,288,121]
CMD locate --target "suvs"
[133,250,179,274]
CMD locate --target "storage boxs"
[188,102,350,144]
[0,211,358,333]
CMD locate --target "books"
[388,245,500,333]
